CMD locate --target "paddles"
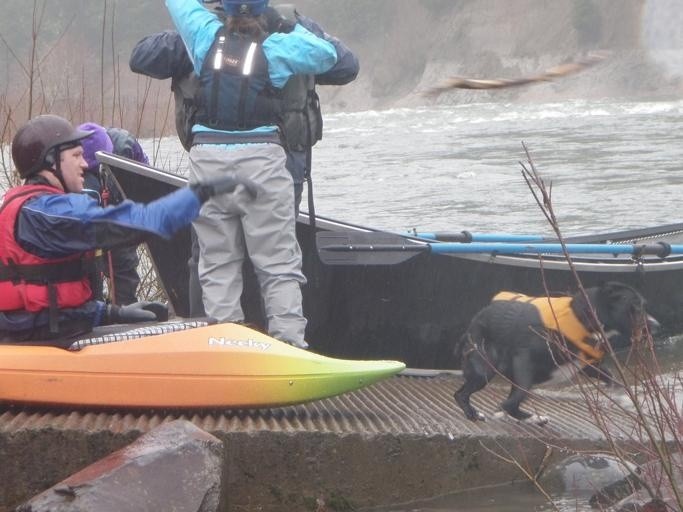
[315,233,683,266]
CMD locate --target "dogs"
[450,281,661,426]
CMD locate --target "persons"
[75,118,150,307]
[0,115,261,340]
[128,1,362,352]
[162,0,335,348]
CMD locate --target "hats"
[77,122,114,171]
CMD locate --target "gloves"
[190,171,272,203]
[103,298,171,322]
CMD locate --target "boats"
[93,156,682,382]
[0,302,413,416]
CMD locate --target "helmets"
[11,114,92,180]
